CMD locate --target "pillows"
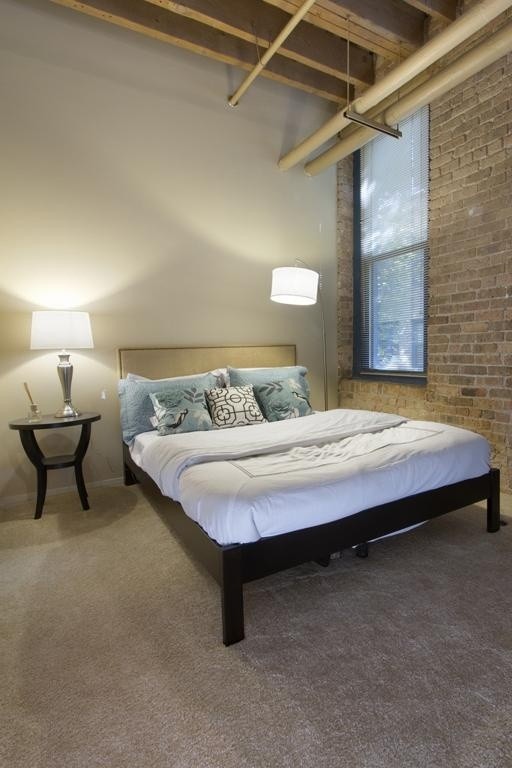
[148,385,213,436]
[252,376,314,422]
[117,368,227,446]
[229,365,310,417]
[204,383,266,429]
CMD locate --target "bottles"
[29,404,41,423]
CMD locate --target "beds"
[119,343,501,646]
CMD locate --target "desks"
[8,412,101,519]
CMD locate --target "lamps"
[30,311,95,418]
[269,258,328,411]
